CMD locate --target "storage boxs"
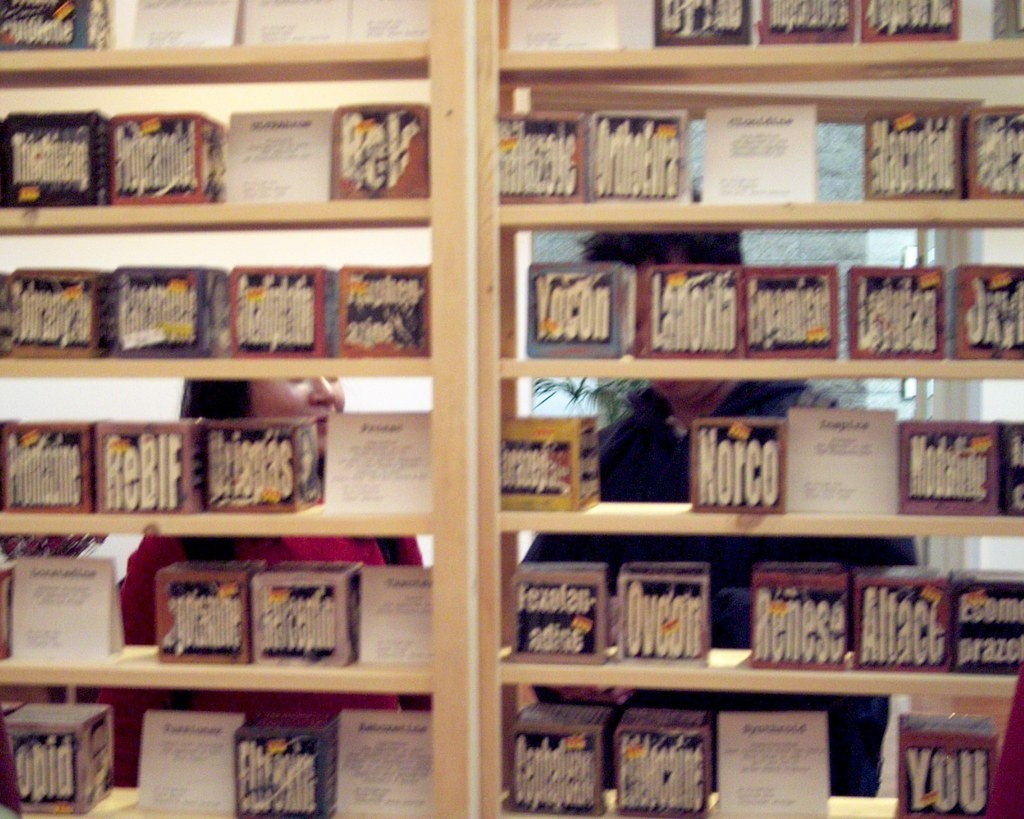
[0,0,1024,819]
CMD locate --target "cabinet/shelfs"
[0,0,1024,819]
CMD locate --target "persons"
[97,380,430,789]
[514,229,915,796]
